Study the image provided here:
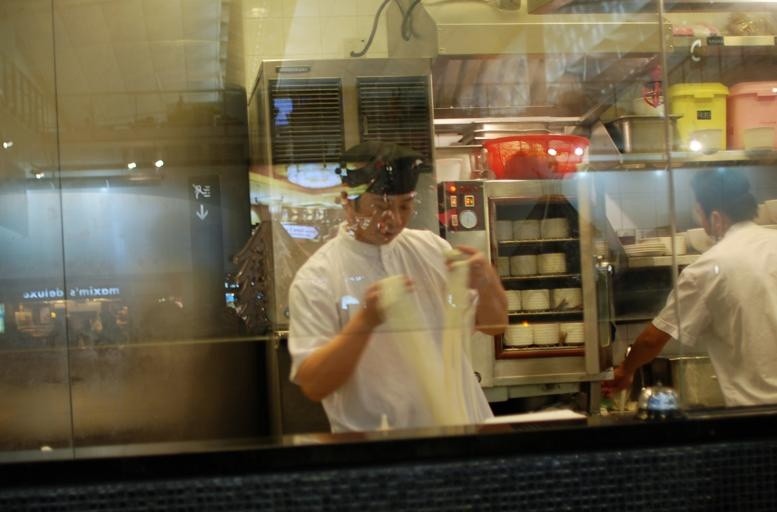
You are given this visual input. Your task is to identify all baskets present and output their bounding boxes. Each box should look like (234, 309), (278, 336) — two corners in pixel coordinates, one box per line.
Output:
(482, 135), (589, 181)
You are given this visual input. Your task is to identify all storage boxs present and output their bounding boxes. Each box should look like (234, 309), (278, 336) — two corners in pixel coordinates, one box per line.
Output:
(728, 79), (777, 150)
(667, 356), (726, 410)
(668, 81), (731, 151)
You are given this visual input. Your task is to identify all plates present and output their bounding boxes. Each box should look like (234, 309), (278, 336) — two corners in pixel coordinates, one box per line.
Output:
(622, 235), (687, 257)
(502, 321), (585, 348)
(492, 217), (572, 241)
(496, 252), (571, 277)
(505, 287), (583, 313)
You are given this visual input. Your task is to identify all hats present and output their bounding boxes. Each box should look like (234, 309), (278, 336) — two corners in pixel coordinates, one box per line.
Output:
(337, 142), (425, 195)
(690, 170), (750, 206)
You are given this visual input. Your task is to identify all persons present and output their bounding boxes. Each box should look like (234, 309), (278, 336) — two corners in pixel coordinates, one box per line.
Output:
(601, 169), (777, 409)
(287, 140), (509, 433)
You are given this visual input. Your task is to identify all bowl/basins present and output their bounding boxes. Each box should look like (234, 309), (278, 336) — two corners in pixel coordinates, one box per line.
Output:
(689, 128), (723, 154)
(674, 228), (718, 255)
(752, 199), (777, 225)
(741, 126), (776, 152)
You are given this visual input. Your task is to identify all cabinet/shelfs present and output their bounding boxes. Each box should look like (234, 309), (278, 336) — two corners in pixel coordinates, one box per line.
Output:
(589, 35), (777, 269)
